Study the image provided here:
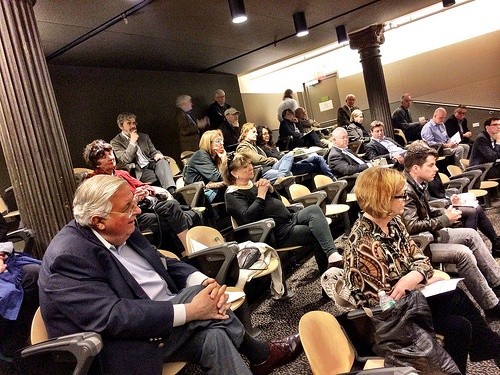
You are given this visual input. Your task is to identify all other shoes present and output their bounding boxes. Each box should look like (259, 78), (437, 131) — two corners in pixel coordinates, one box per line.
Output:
(469, 331), (500, 368)
(491, 284), (500, 299)
(492, 241), (500, 258)
(485, 301), (500, 323)
(327, 258), (344, 270)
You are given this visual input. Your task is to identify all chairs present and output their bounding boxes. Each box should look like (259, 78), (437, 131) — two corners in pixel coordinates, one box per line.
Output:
(1, 125), (500, 375)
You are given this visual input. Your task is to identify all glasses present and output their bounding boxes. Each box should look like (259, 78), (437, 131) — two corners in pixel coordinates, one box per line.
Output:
(102, 195), (139, 218)
(247, 131), (257, 133)
(391, 190), (407, 200)
(402, 100), (413, 102)
(456, 111), (466, 115)
(489, 124), (500, 126)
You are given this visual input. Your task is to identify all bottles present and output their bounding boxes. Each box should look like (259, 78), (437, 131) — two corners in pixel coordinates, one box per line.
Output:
(378, 290), (395, 313)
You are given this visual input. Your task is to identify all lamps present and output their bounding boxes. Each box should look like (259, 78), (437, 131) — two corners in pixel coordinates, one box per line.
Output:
(228, 0), (247, 24)
(442, 0), (455, 8)
(293, 11), (309, 38)
(336, 25), (349, 45)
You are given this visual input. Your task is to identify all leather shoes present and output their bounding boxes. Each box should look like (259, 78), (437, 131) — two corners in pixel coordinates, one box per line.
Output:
(249, 334), (304, 375)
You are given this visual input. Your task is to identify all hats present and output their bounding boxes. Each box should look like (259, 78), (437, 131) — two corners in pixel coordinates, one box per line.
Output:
(224, 108), (241, 116)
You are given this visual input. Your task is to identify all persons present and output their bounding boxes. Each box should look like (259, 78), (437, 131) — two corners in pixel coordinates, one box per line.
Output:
(83, 139), (190, 256)
(182, 130), (230, 203)
(344, 167), (499, 375)
(255, 125), (338, 182)
(275, 87), (500, 176)
(0, 213), (43, 362)
(39, 175), (305, 375)
(109, 114), (175, 195)
(236, 121), (297, 201)
(225, 151), (345, 277)
(327, 127), (375, 193)
(222, 107), (244, 152)
(173, 95), (207, 152)
(402, 141), (500, 323)
(206, 89), (231, 130)
